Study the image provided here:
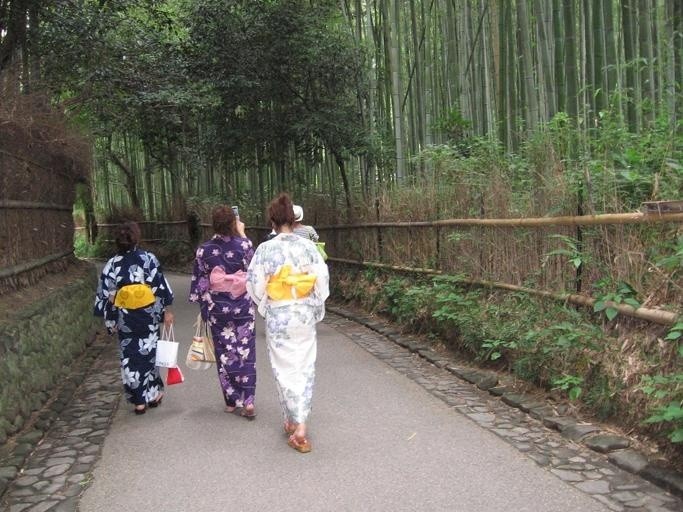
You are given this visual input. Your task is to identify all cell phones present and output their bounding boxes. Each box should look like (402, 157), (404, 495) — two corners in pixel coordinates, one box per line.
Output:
(231, 206), (239, 217)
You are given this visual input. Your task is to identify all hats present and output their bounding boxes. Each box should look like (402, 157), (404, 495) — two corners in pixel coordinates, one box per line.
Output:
(292, 205), (303, 222)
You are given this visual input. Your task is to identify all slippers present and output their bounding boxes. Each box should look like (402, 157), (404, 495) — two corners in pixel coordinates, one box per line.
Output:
(240, 409), (257, 417)
(288, 436), (311, 453)
(136, 395), (163, 414)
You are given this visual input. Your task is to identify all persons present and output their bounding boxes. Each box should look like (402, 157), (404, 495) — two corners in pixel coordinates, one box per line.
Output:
(293, 205), (319, 243)
(245, 191), (330, 453)
(188, 204), (257, 417)
(92, 220), (175, 414)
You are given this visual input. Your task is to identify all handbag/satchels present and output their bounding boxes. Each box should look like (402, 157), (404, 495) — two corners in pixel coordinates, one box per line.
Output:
(186, 337), (215, 370)
(155, 340), (184, 386)
(314, 242), (327, 261)
